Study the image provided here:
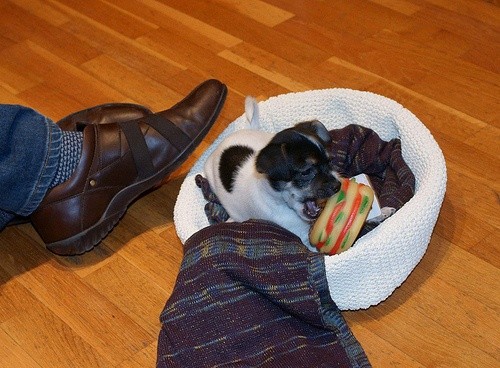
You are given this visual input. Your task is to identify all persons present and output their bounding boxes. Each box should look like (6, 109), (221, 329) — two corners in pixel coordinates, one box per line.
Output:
(0, 79), (228, 255)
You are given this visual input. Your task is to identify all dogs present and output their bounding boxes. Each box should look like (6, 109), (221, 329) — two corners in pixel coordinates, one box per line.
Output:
(205, 120), (341, 253)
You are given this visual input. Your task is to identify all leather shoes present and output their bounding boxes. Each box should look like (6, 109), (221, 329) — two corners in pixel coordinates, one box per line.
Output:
(7, 79), (228, 257)
